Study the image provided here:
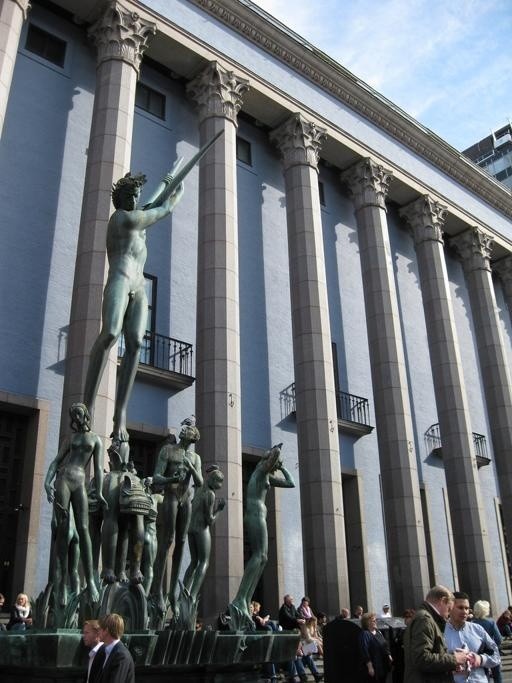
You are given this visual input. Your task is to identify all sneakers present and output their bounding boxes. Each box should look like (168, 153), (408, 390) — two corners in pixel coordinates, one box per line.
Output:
(315, 675), (323, 683)
(300, 675), (307, 683)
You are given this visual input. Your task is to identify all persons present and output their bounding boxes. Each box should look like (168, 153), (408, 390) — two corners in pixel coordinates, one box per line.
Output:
(67, 170), (188, 441)
(94, 612), (137, 682)
(247, 583), (512, 682)
(79, 618), (107, 682)
(40, 403), (298, 634)
(0, 593), (8, 631)
(4, 592), (33, 631)
